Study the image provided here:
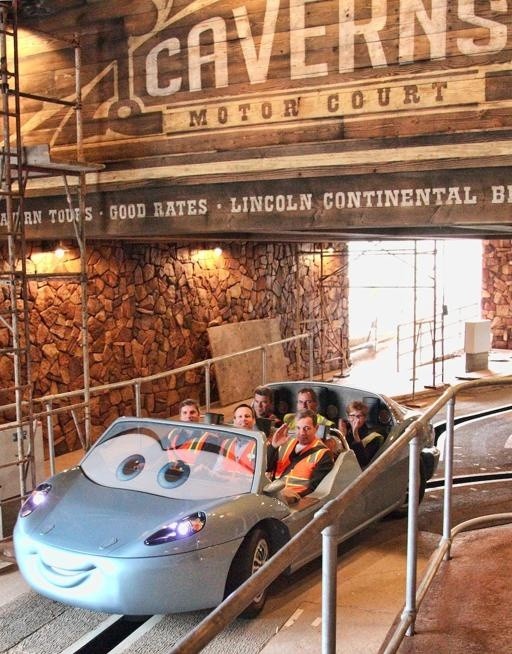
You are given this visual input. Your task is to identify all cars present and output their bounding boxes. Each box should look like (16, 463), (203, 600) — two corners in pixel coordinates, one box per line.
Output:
(14, 380), (440, 620)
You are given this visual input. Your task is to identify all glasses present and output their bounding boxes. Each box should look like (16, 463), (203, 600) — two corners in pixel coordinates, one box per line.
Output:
(348, 414), (366, 419)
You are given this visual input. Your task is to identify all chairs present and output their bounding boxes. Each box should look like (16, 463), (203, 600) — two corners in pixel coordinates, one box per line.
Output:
(266, 383), (283, 416)
(315, 423), (330, 444)
(203, 411), (229, 437)
(359, 394), (385, 438)
(255, 415), (278, 466)
(310, 382), (334, 421)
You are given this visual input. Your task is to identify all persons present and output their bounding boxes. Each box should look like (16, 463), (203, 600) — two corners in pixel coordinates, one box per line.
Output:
(161, 398), (219, 470)
(337, 401), (384, 469)
(287, 388), (338, 437)
(263, 409), (334, 504)
(252, 383), (283, 428)
(194, 404), (274, 482)
